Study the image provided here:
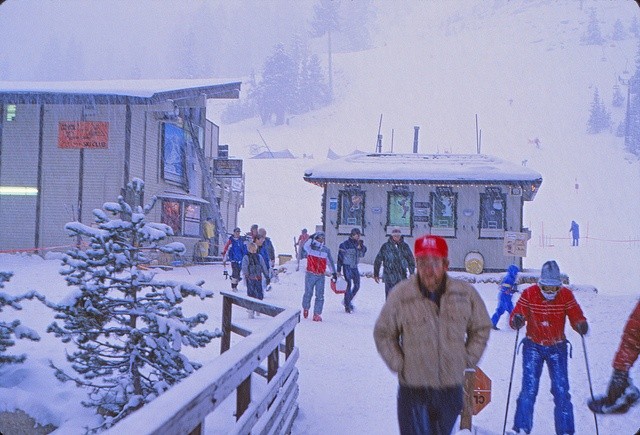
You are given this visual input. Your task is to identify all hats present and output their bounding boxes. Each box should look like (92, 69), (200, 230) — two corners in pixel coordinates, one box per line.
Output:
(414, 236), (448, 258)
(538, 261), (561, 286)
(312, 231), (325, 248)
(351, 229), (360, 235)
(391, 226), (401, 235)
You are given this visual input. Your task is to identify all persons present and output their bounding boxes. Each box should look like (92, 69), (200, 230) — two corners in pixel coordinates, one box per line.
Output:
(202, 217), (215, 256)
(221, 228), (247, 292)
(242, 243), (270, 316)
(337, 228), (367, 314)
(302, 231), (337, 322)
(373, 235), (492, 435)
(569, 221), (580, 246)
(252, 233), (269, 273)
(490, 265), (520, 330)
(602, 296), (640, 435)
(509, 261), (588, 435)
(374, 226), (416, 301)
(244, 224), (258, 243)
(296, 228), (309, 260)
(259, 228), (275, 291)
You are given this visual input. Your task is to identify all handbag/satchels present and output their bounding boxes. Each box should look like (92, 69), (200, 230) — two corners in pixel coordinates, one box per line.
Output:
(330, 276), (347, 293)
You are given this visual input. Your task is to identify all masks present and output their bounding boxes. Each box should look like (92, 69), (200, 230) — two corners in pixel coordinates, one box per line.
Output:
(541, 288), (560, 300)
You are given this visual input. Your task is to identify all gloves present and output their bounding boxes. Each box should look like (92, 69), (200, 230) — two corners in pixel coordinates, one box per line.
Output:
(609, 369), (629, 405)
(331, 273), (337, 284)
(578, 322), (588, 334)
(310, 234), (316, 239)
(512, 314), (525, 329)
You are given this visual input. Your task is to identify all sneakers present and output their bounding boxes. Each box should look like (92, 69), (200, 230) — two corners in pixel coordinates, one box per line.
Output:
(304, 310), (308, 318)
(343, 300), (353, 309)
(345, 307), (352, 312)
(313, 315), (322, 321)
(506, 426), (525, 435)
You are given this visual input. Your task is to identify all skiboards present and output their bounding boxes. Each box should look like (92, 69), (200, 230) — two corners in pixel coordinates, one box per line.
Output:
(294, 235), (301, 272)
